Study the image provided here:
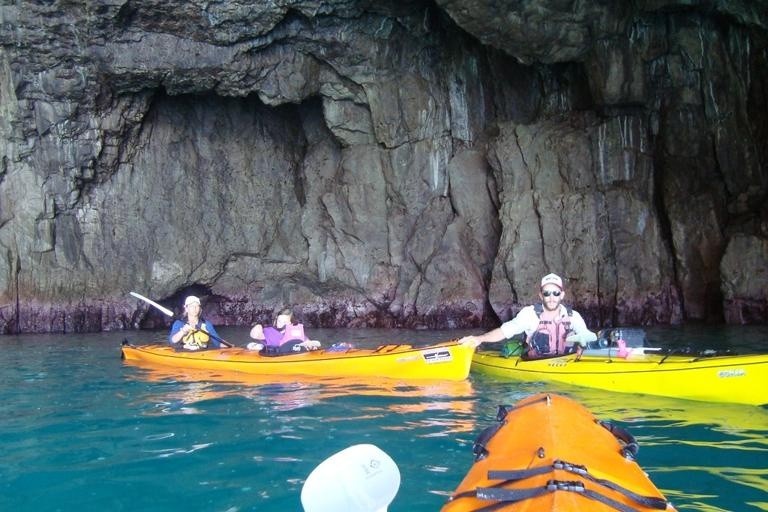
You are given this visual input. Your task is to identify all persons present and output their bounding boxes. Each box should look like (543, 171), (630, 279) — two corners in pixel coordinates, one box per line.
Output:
(457, 271), (596, 360)
(166, 295), (235, 350)
(250, 379), (318, 414)
(248, 309), (322, 355)
(127, 378), (240, 415)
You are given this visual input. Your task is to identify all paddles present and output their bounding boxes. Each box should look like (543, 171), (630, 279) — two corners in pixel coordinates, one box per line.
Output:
(302, 443), (400, 511)
(129, 291), (235, 348)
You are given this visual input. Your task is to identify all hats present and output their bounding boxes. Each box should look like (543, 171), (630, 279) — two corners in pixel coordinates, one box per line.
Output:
(541, 274), (563, 291)
(184, 296), (200, 307)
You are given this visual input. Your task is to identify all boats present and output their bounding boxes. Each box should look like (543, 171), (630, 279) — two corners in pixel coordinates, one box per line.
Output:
(119, 336), (478, 381)
(470, 349), (768, 408)
(438, 389), (676, 511)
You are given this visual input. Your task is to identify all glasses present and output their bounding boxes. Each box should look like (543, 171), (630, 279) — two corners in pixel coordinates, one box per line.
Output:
(543, 291), (560, 296)
